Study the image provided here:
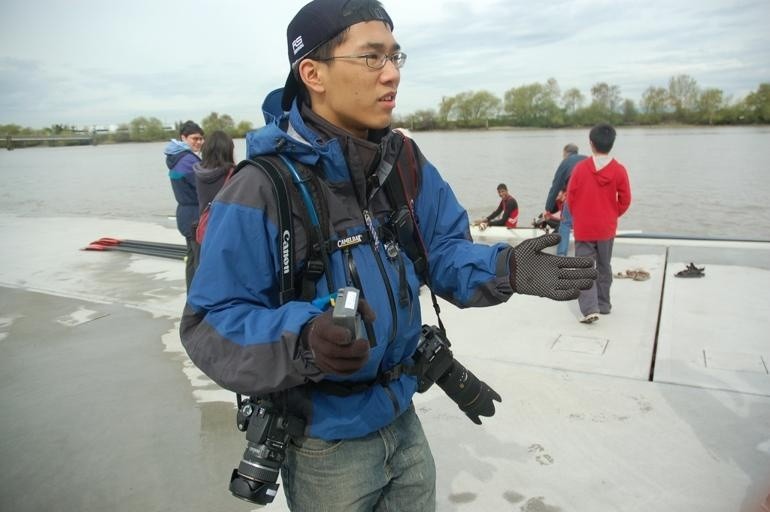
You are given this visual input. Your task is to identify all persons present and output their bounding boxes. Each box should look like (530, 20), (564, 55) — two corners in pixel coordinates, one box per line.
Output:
(163, 118), (208, 300)
(566, 124), (633, 324)
(176, 1), (600, 512)
(530, 190), (564, 232)
(543, 140), (587, 257)
(472, 184), (519, 231)
(190, 129), (239, 246)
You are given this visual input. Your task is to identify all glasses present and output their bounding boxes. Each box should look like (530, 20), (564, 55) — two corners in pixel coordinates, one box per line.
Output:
(308, 52), (406, 70)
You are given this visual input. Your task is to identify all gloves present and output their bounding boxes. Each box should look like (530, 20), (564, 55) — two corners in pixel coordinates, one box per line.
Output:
(300, 299), (376, 377)
(508, 234), (598, 301)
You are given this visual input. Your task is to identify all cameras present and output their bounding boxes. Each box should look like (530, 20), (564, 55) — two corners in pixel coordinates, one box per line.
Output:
(228, 399), (307, 505)
(332, 286), (362, 343)
(416, 324), (501, 425)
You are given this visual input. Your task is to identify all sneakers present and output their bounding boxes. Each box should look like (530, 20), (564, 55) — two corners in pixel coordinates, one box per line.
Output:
(579, 312), (600, 324)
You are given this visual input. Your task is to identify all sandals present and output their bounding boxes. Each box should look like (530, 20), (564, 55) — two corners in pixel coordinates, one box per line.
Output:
(633, 271), (650, 281)
(674, 263), (705, 278)
(613, 270), (637, 278)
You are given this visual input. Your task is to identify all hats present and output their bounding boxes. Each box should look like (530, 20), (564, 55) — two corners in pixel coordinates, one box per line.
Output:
(281, 0), (394, 112)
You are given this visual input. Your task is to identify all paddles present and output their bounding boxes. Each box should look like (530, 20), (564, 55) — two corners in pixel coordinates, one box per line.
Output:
(82, 237), (189, 262)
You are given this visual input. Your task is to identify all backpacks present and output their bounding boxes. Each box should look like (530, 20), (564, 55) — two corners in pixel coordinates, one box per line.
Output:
(187, 130), (423, 418)
